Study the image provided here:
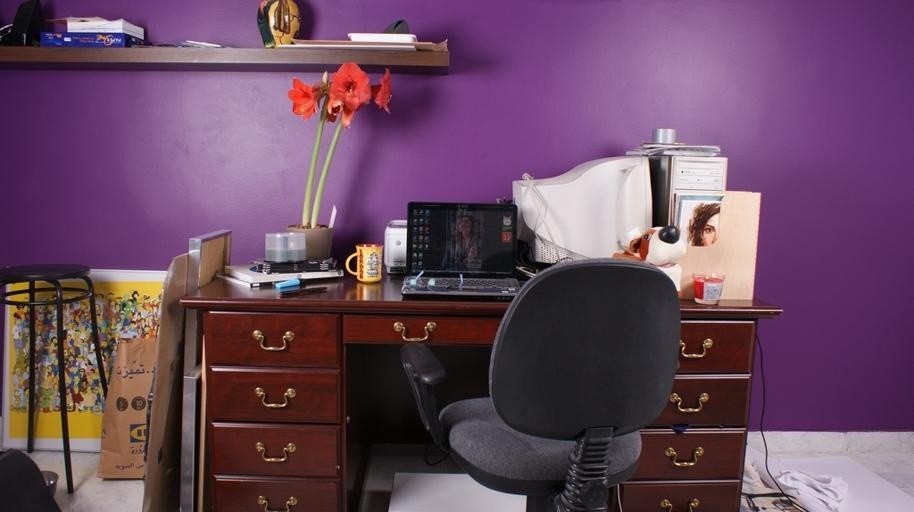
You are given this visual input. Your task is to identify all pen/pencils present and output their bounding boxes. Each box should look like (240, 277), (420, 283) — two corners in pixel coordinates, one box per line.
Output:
(278, 286), (330, 295)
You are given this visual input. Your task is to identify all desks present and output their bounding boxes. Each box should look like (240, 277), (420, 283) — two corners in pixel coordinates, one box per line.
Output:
(177, 257), (785, 511)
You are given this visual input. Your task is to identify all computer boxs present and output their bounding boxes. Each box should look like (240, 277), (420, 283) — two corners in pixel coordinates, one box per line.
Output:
(648, 155), (728, 227)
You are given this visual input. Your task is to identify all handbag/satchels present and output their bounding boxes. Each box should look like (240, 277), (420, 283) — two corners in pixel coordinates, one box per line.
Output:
(97, 338), (158, 481)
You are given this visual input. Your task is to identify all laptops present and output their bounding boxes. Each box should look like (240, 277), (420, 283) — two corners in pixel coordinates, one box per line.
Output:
(400, 201), (521, 301)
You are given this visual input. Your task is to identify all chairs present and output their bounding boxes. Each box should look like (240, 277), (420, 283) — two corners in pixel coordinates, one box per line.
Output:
(399, 260), (684, 508)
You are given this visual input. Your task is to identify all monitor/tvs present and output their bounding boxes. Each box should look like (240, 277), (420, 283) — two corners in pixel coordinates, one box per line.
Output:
(512, 155), (653, 264)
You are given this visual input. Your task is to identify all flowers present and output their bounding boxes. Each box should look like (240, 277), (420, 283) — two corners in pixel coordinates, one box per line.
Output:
(282, 60), (393, 226)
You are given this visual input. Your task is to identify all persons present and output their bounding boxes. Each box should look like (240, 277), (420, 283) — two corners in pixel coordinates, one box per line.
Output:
(12, 290), (162, 413)
(687, 200), (721, 247)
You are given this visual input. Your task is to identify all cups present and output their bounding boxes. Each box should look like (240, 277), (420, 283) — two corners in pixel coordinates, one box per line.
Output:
(346, 245), (384, 280)
(695, 270), (726, 305)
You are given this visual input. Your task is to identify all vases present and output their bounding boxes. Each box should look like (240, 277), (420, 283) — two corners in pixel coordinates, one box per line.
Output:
(290, 223), (334, 261)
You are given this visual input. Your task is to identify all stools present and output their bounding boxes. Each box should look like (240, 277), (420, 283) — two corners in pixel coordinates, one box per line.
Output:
(2, 262), (114, 495)
